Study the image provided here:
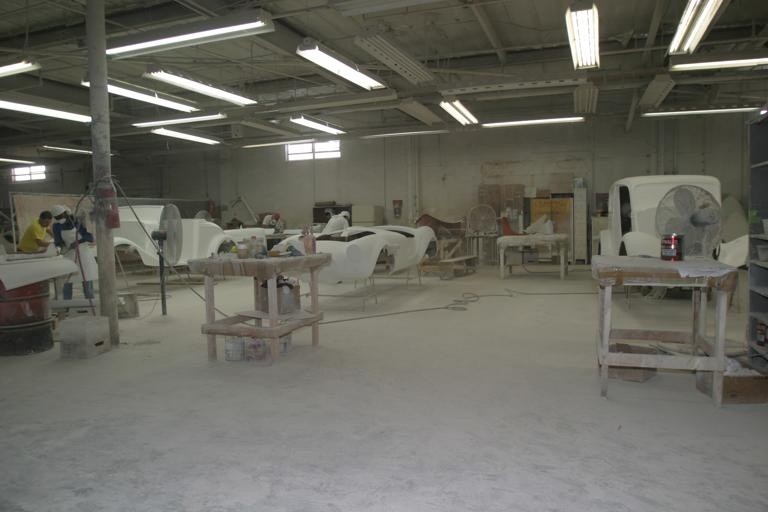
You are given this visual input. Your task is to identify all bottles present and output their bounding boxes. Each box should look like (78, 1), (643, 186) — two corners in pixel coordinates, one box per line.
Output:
(756, 325), (766, 346)
(238, 241), (248, 258)
(250, 236), (258, 258)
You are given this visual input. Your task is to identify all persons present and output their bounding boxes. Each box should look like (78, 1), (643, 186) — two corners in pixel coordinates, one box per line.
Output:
(17, 210), (53, 253)
(51, 204), (98, 299)
(322, 211), (350, 233)
(262, 213), (284, 229)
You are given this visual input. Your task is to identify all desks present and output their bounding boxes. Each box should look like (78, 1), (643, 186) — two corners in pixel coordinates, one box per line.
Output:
(590, 258), (736, 401)
(186, 251), (332, 362)
(496, 234), (570, 280)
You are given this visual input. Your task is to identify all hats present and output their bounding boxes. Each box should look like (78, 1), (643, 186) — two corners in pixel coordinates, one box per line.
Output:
(338, 210), (350, 218)
(51, 205), (65, 217)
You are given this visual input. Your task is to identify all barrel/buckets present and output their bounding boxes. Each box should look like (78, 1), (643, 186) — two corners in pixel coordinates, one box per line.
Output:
(257, 283), (301, 316)
(0, 268), (56, 357)
(224, 334), (244, 361)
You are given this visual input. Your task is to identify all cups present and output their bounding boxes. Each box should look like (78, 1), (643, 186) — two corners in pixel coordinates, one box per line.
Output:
(756, 245), (768, 263)
(761, 218), (768, 236)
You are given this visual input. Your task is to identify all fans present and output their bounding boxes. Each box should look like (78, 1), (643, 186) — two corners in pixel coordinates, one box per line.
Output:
(150, 202), (183, 329)
(655, 184), (723, 353)
(470, 204), (497, 264)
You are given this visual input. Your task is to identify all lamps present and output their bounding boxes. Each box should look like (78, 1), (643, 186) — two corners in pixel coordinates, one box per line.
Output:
(0, 7), (277, 163)
(569, 81), (600, 117)
(287, 112), (349, 139)
(668, 47), (767, 73)
(293, 35), (386, 97)
(399, 95), (445, 130)
(637, 72), (675, 111)
(481, 110), (588, 130)
(356, 123), (455, 138)
(352, 25), (437, 87)
(640, 100), (762, 120)
(665, 1), (728, 59)
(563, 0), (606, 73)
(436, 92), (480, 128)
(238, 115), (303, 142)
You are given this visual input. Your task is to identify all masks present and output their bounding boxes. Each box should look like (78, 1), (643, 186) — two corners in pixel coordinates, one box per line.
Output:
(57, 218), (67, 224)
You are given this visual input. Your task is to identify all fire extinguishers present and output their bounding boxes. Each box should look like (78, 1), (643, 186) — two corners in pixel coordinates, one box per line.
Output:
(100, 174), (121, 229)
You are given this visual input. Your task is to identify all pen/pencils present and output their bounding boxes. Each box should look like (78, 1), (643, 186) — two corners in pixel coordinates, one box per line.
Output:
(303, 224), (315, 234)
